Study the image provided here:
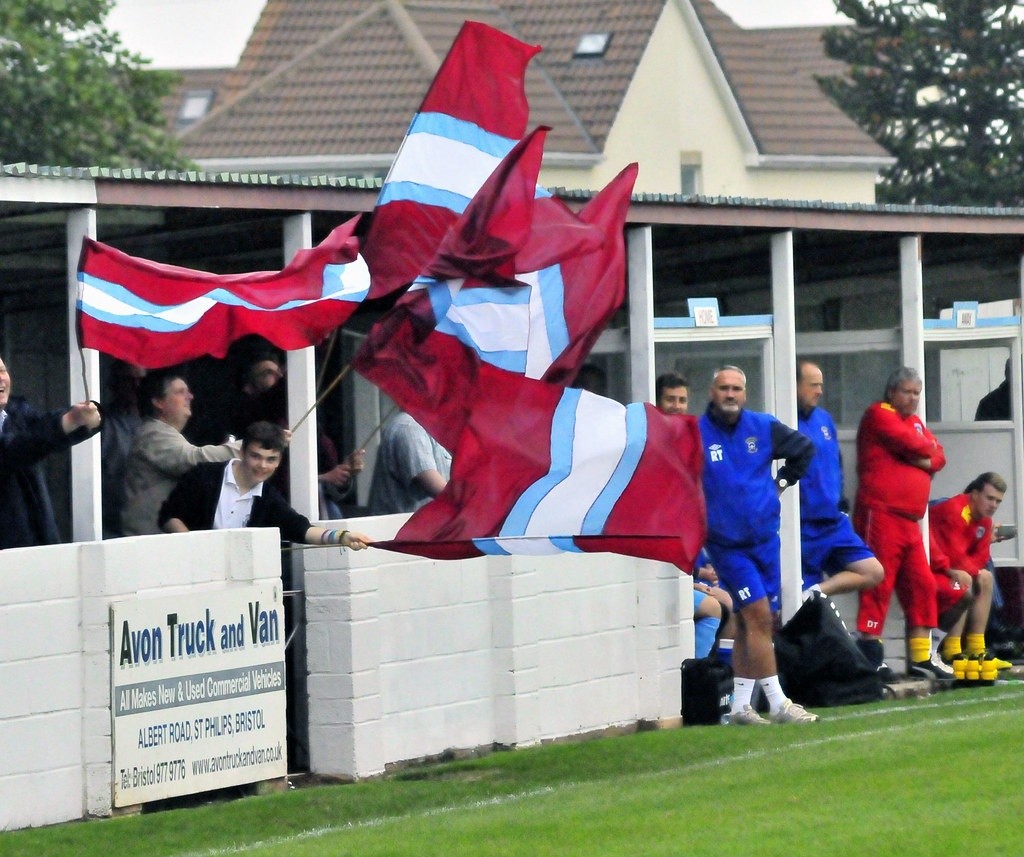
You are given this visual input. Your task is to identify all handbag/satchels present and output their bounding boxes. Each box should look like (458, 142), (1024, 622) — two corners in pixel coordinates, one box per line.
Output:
(679, 655), (733, 722)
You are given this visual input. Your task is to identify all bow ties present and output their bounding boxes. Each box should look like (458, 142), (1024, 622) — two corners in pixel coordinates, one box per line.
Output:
(935, 646), (987, 674)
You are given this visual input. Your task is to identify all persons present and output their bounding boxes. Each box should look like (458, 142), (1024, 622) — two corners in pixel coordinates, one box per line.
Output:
(100, 360), (456, 551)
(0, 357), (103, 551)
(973, 354), (1024, 649)
(649, 360), (1012, 727)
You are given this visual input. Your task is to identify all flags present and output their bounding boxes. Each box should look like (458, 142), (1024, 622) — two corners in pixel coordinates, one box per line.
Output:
(341, 21), (706, 575)
(74, 213), (370, 368)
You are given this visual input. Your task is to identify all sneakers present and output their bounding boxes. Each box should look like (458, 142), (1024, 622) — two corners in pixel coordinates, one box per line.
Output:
(768, 700), (818, 724)
(904, 650), (955, 678)
(719, 705), (771, 727)
(865, 657), (901, 684)
(970, 653), (1013, 672)
